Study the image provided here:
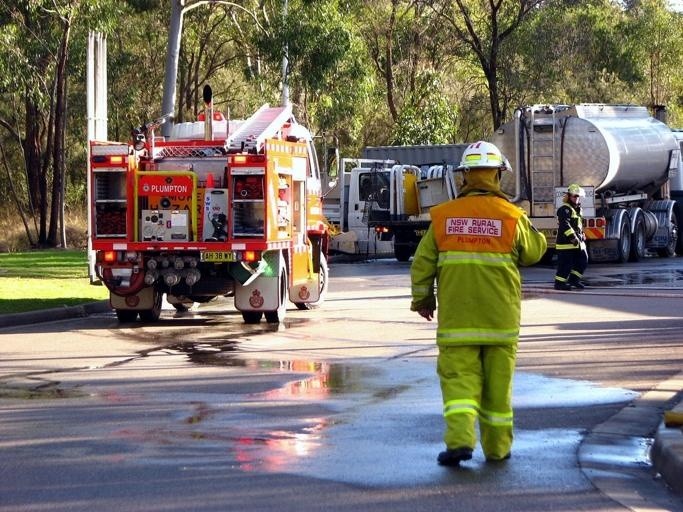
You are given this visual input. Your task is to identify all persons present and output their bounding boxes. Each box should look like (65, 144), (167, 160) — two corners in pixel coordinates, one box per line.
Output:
(408, 140), (549, 464)
(553, 183), (589, 290)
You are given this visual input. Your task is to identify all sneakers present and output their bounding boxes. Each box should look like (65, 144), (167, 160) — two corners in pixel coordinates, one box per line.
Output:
(568, 279), (585, 289)
(486, 450), (511, 464)
(554, 283), (574, 290)
(438, 446), (473, 462)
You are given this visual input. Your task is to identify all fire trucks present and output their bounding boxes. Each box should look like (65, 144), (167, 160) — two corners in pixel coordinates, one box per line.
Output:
(78, 81), (336, 327)
(486, 101), (683, 264)
(320, 143), (475, 263)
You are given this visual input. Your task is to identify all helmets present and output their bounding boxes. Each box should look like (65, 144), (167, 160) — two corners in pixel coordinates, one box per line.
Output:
(452, 140), (503, 171)
(569, 185), (585, 196)
